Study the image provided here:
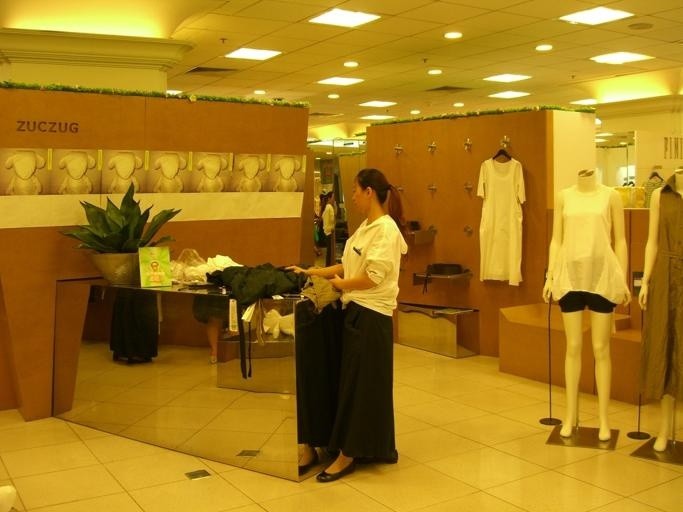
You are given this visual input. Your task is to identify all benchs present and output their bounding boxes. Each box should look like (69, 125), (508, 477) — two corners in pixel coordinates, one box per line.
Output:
(215, 326), (297, 397)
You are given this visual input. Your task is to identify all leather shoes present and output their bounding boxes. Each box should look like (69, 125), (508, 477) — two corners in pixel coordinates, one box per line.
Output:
(316, 459), (355, 482)
(298, 450), (319, 475)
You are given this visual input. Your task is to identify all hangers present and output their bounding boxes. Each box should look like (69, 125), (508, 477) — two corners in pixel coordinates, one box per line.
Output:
(494, 137), (511, 160)
(647, 165), (662, 182)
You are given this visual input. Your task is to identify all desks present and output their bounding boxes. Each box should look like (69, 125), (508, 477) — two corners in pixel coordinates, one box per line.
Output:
(51, 277), (336, 482)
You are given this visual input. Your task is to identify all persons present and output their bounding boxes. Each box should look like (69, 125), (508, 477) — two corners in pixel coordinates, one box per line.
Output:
(542, 169), (632, 442)
(294, 298), (342, 475)
(638, 168), (683, 453)
(321, 192), (335, 267)
(285, 168), (410, 483)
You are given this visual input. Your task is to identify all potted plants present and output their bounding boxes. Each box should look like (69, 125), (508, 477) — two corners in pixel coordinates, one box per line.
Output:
(57, 182), (182, 286)
